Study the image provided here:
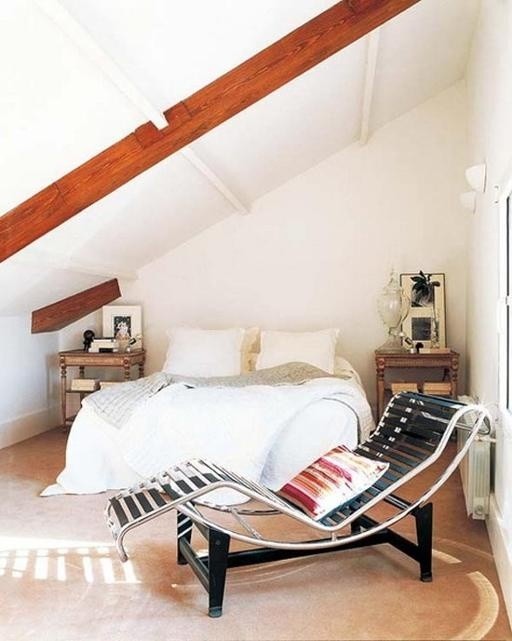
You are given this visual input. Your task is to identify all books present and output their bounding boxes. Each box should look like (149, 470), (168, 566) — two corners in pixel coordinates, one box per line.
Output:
(387, 379), (452, 396)
(419, 348), (450, 354)
(71, 377), (126, 392)
(88, 337), (131, 353)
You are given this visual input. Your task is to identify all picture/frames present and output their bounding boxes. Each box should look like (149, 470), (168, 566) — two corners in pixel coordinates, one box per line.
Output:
(102, 305), (143, 354)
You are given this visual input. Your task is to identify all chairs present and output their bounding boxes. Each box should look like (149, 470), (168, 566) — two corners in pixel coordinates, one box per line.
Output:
(102, 389), (487, 620)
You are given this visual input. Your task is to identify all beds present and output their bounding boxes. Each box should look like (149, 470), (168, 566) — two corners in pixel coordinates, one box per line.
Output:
(36, 347), (375, 506)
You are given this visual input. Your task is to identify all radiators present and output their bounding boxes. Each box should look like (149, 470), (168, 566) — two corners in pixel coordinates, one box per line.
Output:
(457, 396), (492, 523)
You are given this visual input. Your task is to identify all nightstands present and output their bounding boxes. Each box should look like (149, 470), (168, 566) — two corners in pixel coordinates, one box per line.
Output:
(374, 345), (458, 435)
(58, 349), (148, 438)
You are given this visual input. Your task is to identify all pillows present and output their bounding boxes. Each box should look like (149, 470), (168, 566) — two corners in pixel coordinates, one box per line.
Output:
(257, 329), (340, 376)
(159, 327), (256, 380)
(276, 443), (390, 520)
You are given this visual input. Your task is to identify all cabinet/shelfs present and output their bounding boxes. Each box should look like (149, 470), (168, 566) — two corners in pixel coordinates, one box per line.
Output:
(399, 272), (446, 350)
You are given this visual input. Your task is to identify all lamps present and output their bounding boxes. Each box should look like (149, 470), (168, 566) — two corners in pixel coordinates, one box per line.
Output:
(457, 189), (477, 214)
(463, 162), (487, 195)
